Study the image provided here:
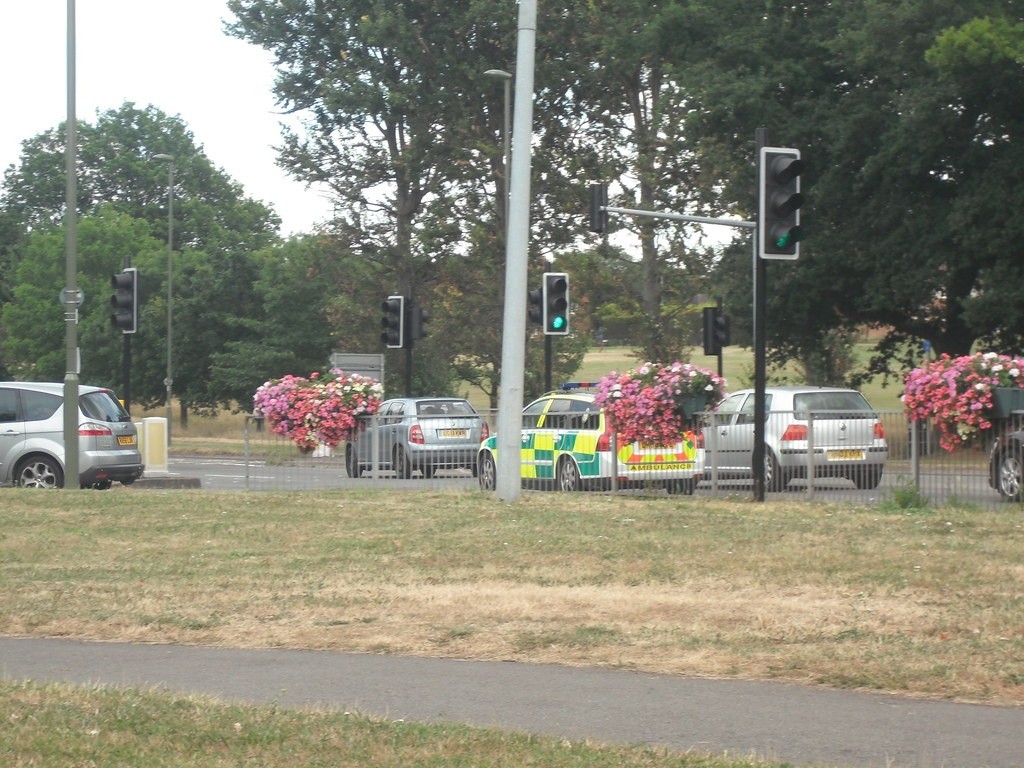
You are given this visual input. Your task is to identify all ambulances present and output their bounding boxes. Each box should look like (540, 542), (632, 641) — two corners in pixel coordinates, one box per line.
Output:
(475, 382), (706, 492)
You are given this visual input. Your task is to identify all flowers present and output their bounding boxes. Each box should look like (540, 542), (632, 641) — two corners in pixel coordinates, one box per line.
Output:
(899, 350), (1024, 453)
(593, 358), (727, 450)
(252, 367), (386, 454)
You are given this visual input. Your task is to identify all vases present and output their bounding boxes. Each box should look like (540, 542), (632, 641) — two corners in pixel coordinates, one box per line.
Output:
(979, 386), (1024, 419)
(671, 393), (707, 419)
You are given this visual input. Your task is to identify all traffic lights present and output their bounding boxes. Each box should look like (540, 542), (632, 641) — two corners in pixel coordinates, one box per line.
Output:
(543, 272), (569, 335)
(757, 146), (805, 260)
(110, 267), (138, 335)
(714, 314), (730, 346)
(381, 296), (405, 349)
(527, 288), (543, 326)
(589, 183), (608, 233)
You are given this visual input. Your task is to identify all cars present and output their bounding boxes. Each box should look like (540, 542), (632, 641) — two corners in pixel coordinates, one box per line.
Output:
(700, 386), (889, 492)
(345, 397), (489, 479)
(0, 381), (145, 489)
(988, 424), (1024, 502)
